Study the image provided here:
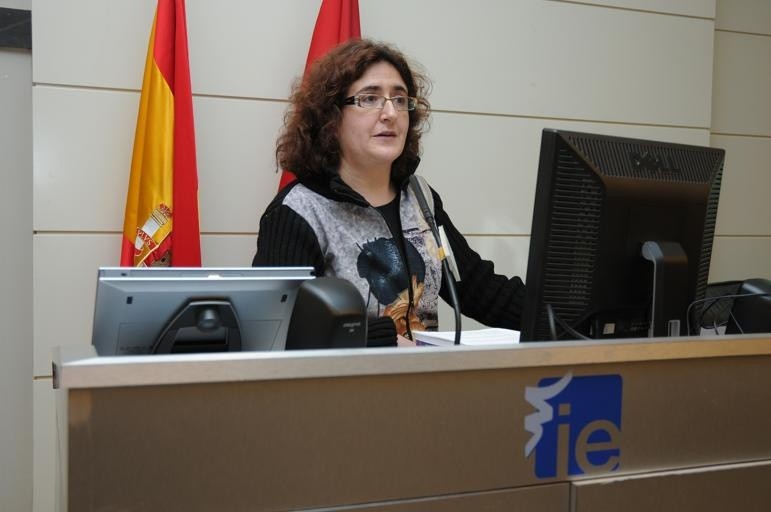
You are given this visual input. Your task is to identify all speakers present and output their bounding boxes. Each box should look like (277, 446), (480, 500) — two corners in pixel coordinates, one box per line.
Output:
(285, 278), (366, 349)
(728, 278), (771, 334)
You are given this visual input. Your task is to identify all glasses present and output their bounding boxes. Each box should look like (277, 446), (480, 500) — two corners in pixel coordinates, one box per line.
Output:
(335, 92), (418, 113)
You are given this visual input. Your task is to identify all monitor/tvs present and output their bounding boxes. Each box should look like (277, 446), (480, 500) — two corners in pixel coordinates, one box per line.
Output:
(518, 129), (726, 345)
(90, 265), (312, 358)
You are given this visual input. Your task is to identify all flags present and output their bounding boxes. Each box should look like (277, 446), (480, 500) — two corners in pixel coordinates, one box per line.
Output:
(277, 1), (360, 193)
(118, 0), (201, 267)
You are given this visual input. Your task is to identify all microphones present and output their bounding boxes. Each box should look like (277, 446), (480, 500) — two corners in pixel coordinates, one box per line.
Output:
(410, 173), (461, 344)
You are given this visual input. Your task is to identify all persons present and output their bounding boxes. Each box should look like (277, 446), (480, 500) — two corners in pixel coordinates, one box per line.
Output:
(250, 38), (525, 341)
